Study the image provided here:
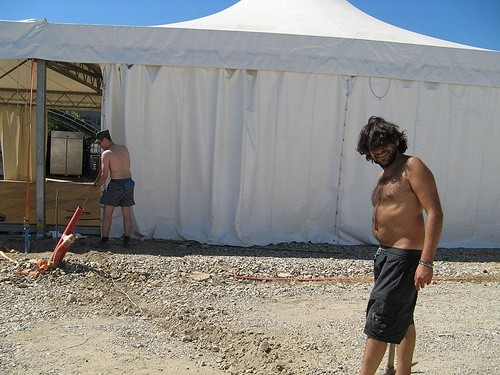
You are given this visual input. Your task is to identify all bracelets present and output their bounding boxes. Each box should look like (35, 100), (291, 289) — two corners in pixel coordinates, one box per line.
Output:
(419, 260), (436, 269)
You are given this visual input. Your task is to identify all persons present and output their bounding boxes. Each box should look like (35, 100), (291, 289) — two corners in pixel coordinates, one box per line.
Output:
(90, 127), (137, 244)
(353, 116), (446, 375)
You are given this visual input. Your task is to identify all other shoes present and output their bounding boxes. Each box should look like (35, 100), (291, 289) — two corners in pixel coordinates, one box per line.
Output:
(120, 234), (130, 246)
(96, 236), (108, 244)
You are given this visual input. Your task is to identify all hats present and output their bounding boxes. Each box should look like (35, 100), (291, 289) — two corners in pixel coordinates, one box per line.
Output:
(94, 129), (109, 143)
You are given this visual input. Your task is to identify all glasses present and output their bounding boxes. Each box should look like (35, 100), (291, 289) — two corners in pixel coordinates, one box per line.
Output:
(98, 140), (102, 145)
